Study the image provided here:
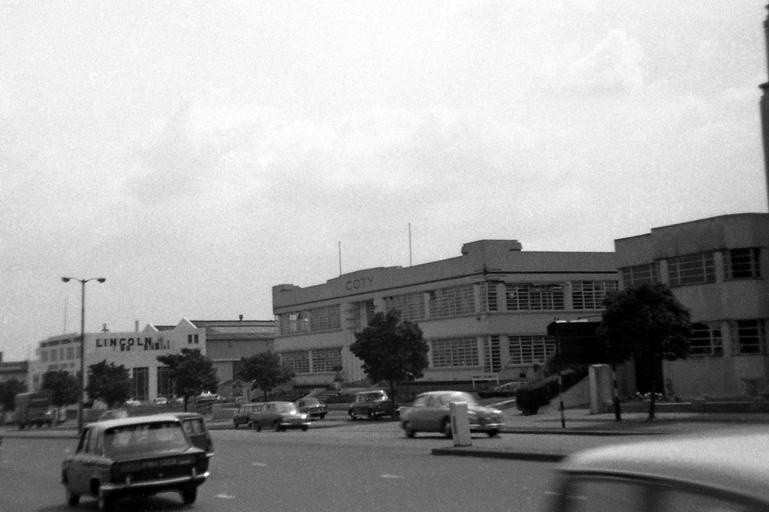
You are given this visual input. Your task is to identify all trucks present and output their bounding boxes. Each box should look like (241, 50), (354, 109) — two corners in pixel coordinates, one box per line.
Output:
(14, 391), (54, 429)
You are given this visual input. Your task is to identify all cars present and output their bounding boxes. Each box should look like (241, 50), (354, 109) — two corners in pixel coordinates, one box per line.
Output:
(233, 397), (327, 432)
(400, 391), (503, 438)
(349, 390), (391, 418)
(479, 381), (527, 398)
(60, 390), (227, 510)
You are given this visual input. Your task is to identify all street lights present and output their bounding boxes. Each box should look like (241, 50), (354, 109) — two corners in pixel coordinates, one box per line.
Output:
(62, 277), (105, 432)
